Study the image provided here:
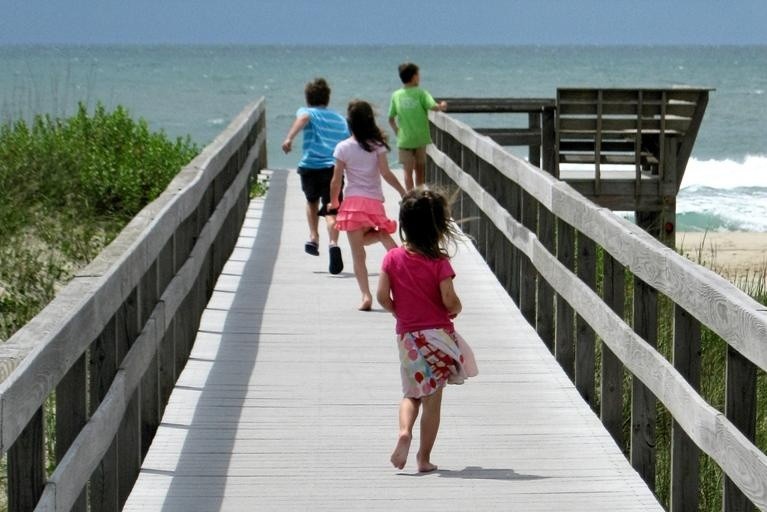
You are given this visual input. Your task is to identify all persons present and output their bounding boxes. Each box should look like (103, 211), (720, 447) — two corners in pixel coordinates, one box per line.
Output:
(389, 62), (448, 190)
(376, 184), (479, 472)
(282, 78), (351, 275)
(327, 101), (408, 311)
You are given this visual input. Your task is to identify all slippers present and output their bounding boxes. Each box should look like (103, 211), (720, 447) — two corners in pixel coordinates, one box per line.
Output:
(304, 241), (319, 256)
(328, 245), (344, 274)
